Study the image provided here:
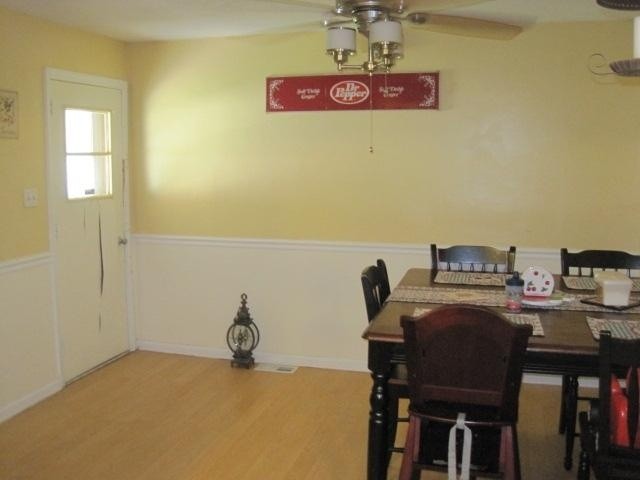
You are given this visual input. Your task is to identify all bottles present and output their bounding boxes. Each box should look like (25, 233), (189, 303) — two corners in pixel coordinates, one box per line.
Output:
(505, 272), (523, 313)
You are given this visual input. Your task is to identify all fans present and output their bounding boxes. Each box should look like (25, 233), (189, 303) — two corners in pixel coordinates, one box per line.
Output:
(223, 0), (524, 45)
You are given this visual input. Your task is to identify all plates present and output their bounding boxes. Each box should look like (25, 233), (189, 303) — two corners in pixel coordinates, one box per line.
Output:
(579, 295), (639, 310)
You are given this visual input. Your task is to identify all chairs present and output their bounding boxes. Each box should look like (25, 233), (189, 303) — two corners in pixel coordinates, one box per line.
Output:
(578, 329), (640, 480)
(362, 259), (414, 454)
(431, 244), (515, 284)
(399, 302), (534, 480)
(559, 247), (639, 471)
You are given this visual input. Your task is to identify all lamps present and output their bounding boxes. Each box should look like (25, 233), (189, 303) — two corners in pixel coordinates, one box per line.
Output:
(586, 15), (639, 77)
(226, 292), (260, 370)
(323, 0), (407, 153)
